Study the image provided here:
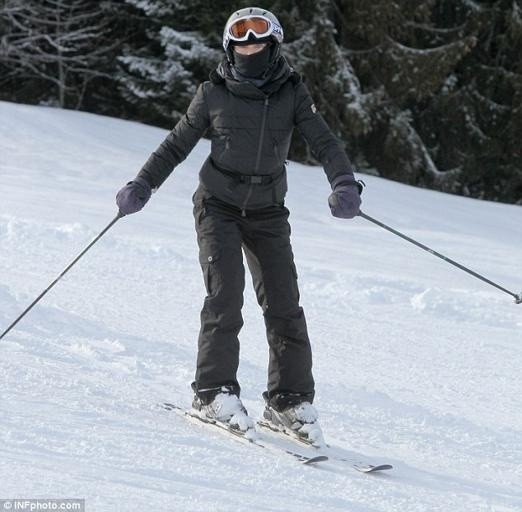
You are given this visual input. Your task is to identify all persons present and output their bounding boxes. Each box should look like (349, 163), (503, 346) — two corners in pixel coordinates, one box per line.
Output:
(116, 7), (365, 447)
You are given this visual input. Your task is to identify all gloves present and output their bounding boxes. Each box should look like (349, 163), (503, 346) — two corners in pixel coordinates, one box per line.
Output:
(327, 182), (363, 219)
(115, 177), (151, 216)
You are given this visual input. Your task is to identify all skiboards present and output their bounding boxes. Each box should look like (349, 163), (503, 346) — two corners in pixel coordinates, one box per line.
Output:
(161, 403), (392, 473)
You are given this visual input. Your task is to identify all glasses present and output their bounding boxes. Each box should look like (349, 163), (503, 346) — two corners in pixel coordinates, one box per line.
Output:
(224, 17), (282, 48)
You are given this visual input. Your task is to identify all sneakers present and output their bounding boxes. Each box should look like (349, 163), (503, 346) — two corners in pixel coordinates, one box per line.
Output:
(264, 397), (326, 448)
(190, 385), (255, 437)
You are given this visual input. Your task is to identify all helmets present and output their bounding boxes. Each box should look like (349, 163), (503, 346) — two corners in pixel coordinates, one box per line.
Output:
(221, 6), (283, 63)
